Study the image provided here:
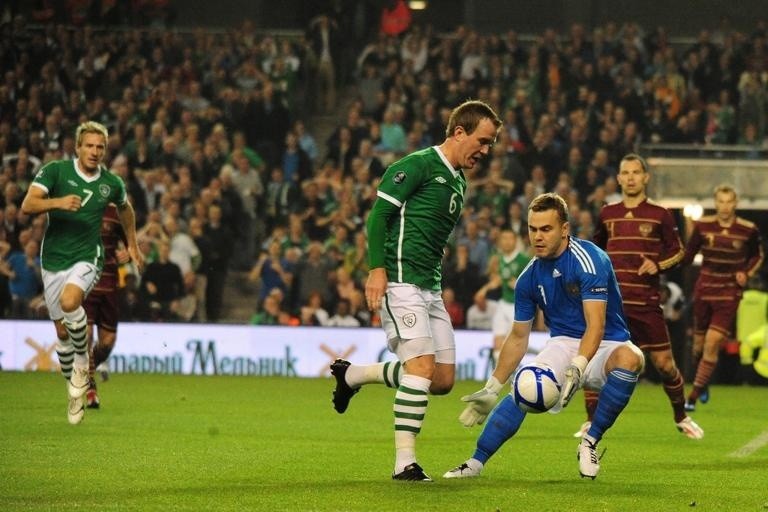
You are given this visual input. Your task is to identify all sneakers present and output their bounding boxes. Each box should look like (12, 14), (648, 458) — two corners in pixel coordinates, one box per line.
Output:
(674, 390), (708, 439)
(442, 463), (479, 479)
(391, 464), (433, 483)
(573, 422), (599, 480)
(67, 362), (99, 425)
(329, 359), (361, 413)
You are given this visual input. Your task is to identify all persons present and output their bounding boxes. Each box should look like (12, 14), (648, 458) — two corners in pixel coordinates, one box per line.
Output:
(442, 192), (646, 480)
(81, 202), (133, 408)
(21, 121), (142, 424)
(573, 154), (703, 440)
(684, 185), (765, 410)
(1, 0), (768, 382)
(329, 100), (503, 483)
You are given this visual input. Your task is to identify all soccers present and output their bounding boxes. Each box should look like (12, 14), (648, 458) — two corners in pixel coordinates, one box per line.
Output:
(512, 363), (560, 412)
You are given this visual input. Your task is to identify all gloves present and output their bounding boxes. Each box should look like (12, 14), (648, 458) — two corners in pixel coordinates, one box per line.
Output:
(458, 376), (505, 428)
(546, 356), (588, 414)
